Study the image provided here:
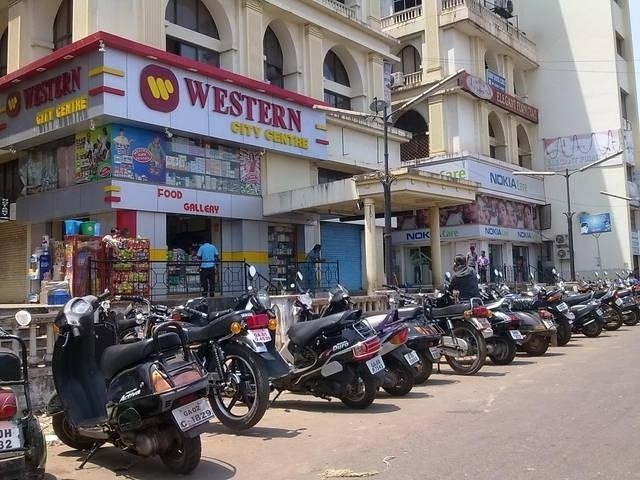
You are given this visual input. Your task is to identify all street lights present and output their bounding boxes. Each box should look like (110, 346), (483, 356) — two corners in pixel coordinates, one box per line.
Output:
(313, 69), (467, 285)
(513, 150), (639, 280)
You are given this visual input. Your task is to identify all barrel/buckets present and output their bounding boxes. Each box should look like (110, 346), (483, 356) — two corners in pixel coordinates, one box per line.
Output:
(64, 219), (81, 235)
(81, 221), (96, 235)
(47, 290), (70, 305)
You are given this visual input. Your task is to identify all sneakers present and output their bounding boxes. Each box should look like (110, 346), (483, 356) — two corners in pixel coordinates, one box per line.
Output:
(202, 292), (214, 297)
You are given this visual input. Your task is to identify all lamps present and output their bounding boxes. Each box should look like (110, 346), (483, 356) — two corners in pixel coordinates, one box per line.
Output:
(164, 128), (173, 138)
(259, 149), (266, 157)
(9, 145), (17, 154)
(98, 39), (106, 53)
(88, 119), (95, 130)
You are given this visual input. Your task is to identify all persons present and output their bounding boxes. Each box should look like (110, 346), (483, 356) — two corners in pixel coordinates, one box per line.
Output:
(148, 135), (166, 174)
(84, 128), (111, 170)
(303, 244), (325, 295)
(466, 246), (478, 269)
(196, 236), (220, 297)
(113, 128), (133, 144)
(101, 227), (121, 242)
(449, 254), (482, 304)
(397, 195), (534, 230)
(477, 250), (489, 284)
(246, 153), (258, 173)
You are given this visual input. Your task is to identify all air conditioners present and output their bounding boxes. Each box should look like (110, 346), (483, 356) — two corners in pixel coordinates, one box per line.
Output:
(390, 71), (404, 87)
(555, 233), (570, 260)
(494, 0), (514, 18)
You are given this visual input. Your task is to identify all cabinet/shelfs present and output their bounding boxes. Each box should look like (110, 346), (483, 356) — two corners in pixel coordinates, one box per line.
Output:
(164, 134), (241, 194)
(106, 238), (150, 301)
(268, 225), (298, 290)
(27, 247), (44, 304)
(168, 247), (202, 294)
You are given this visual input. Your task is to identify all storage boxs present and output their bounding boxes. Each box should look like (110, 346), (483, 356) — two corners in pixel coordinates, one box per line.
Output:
(64, 220), (101, 236)
